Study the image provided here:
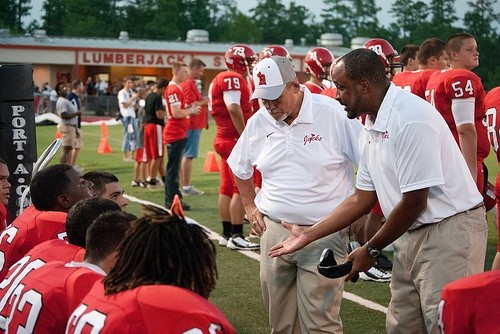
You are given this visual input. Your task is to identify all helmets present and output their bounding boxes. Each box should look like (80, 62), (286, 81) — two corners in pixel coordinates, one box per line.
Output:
(364, 39), (398, 67)
(304, 48), (336, 79)
(224, 45), (258, 73)
(259, 45), (293, 62)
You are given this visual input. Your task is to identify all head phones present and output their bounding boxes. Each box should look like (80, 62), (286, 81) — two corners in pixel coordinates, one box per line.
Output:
(317, 241), (362, 283)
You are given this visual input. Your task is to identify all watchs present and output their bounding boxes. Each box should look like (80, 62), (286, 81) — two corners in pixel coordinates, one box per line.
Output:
(364, 241), (381, 259)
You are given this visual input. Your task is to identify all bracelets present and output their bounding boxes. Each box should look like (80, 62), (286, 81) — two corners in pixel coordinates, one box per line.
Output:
(188, 106), (195, 114)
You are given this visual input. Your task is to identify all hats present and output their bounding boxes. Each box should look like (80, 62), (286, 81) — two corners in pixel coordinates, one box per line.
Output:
(250, 56), (294, 101)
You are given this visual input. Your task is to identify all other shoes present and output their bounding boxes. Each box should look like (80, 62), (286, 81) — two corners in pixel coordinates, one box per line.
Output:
(166, 201), (190, 210)
(147, 181), (165, 192)
(181, 187), (204, 196)
(73, 165), (83, 172)
(124, 157), (136, 162)
(139, 180), (148, 187)
(131, 180), (140, 186)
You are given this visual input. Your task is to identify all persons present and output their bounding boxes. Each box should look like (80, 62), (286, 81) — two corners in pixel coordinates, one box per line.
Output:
(268, 47), (485, 334)
(163, 63), (202, 209)
(68, 80), (85, 172)
(34, 76), (157, 116)
(481, 86), (500, 270)
(206, 33), (496, 282)
(226, 56), (365, 334)
(118, 75), (169, 190)
(181, 60), (210, 195)
(437, 269), (500, 334)
(0, 158), (236, 334)
(55, 82), (81, 167)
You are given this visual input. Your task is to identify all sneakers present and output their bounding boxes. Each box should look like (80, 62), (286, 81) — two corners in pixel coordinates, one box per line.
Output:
(227, 235), (260, 250)
(218, 235), (228, 246)
(359, 266), (392, 282)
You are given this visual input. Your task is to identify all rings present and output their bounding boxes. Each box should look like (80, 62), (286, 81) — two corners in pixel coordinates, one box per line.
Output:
(252, 220), (259, 226)
